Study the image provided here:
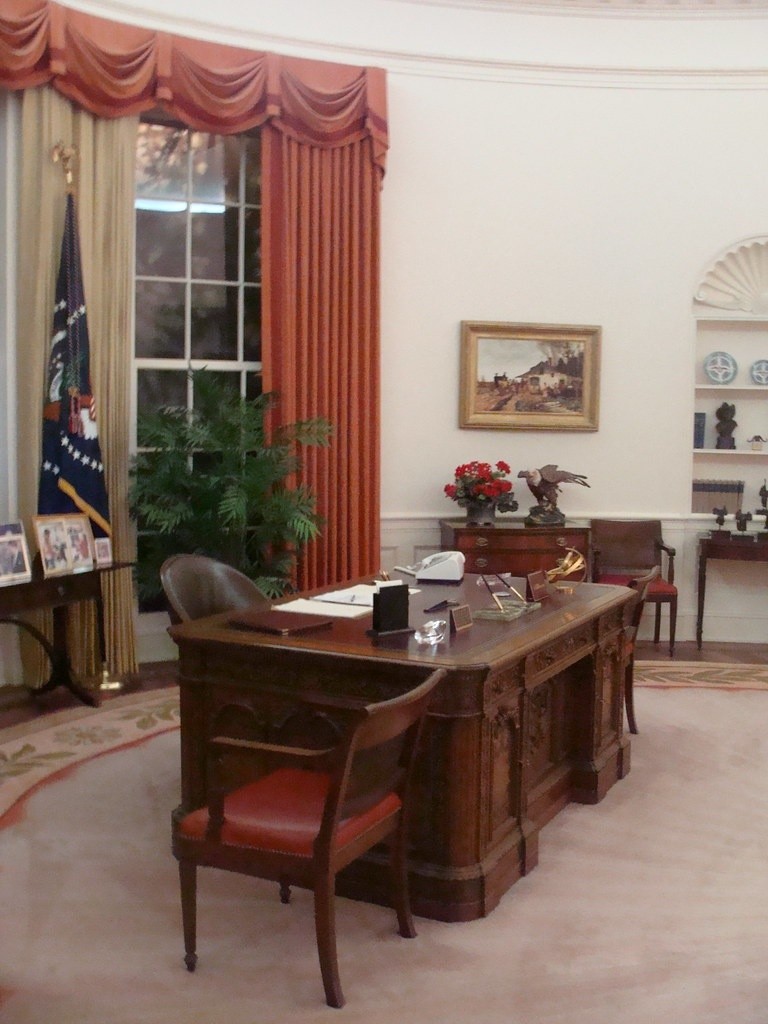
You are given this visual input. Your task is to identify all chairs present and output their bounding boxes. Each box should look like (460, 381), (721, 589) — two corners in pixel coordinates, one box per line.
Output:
(588, 518), (677, 659)
(625, 565), (659, 734)
(174, 668), (446, 1009)
(159, 553), (271, 626)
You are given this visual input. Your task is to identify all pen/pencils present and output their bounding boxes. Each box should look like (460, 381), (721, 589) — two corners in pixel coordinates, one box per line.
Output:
(481, 574), (502, 610)
(351, 594), (356, 602)
(496, 574), (526, 603)
(379, 570), (391, 581)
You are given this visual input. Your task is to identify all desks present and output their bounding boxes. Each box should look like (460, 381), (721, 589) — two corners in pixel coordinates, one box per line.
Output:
(0, 560), (138, 709)
(165, 564), (643, 923)
(695, 531), (768, 653)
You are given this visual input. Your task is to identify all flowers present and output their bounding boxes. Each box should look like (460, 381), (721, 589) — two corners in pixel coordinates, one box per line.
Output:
(444, 460), (519, 514)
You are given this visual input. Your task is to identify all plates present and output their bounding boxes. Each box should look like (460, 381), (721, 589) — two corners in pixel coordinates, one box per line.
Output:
(702, 351), (738, 385)
(750, 360), (768, 385)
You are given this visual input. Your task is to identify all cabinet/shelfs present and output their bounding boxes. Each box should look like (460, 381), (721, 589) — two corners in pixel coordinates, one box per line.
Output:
(691, 385), (768, 518)
(439, 519), (590, 583)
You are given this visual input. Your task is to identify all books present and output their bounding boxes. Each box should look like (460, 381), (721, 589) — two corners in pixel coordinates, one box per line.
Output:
(226, 608), (334, 636)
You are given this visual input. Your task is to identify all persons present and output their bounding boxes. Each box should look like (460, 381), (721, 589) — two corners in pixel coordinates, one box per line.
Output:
(74, 533), (88, 559)
(44, 529), (68, 571)
(6, 540), (26, 573)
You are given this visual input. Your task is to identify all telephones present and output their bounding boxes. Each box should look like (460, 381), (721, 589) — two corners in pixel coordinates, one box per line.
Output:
(415, 550), (466, 585)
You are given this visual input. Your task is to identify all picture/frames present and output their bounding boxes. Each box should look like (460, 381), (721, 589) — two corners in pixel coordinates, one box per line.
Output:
(457, 319), (602, 433)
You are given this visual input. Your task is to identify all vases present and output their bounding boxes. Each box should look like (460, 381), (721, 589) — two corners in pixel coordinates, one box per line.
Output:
(464, 497), (496, 528)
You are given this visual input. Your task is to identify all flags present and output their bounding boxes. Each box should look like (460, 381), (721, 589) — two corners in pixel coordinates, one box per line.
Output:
(36, 193), (112, 566)
